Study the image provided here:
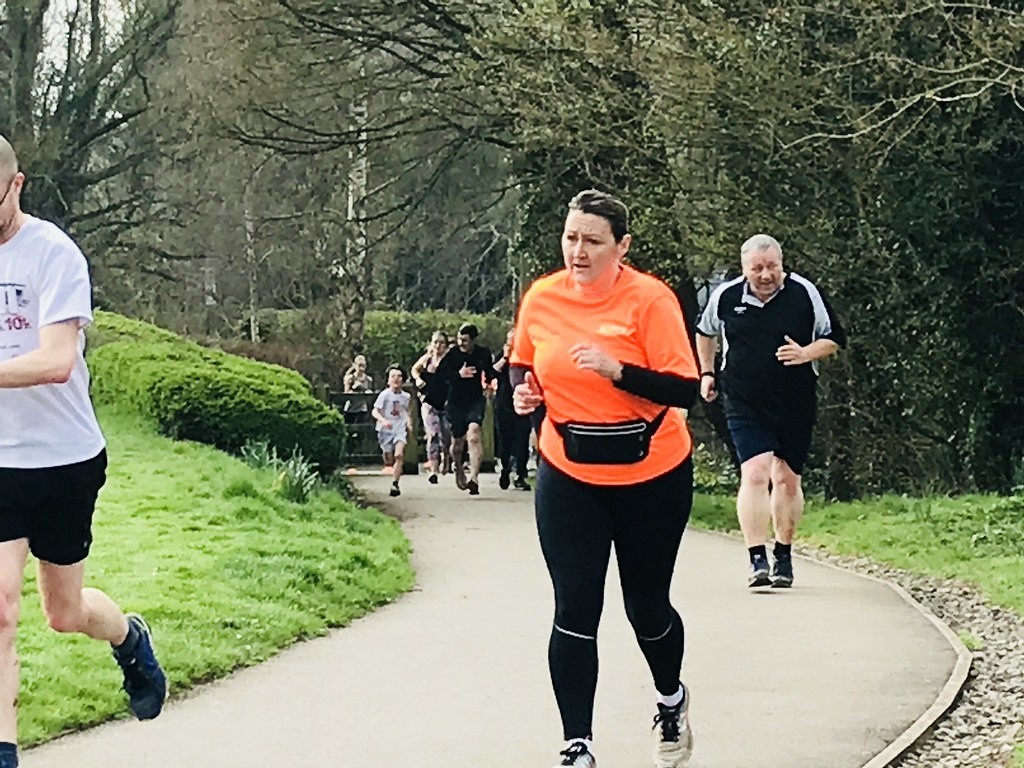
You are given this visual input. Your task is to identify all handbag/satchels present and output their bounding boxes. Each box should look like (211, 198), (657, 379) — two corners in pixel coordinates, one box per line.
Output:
(548, 405), (671, 464)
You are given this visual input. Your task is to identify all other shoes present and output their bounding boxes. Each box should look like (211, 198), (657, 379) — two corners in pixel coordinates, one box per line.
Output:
(389, 486), (401, 496)
(513, 477), (532, 491)
(454, 468), (468, 491)
(427, 473), (439, 484)
(500, 469), (512, 489)
(466, 479), (480, 495)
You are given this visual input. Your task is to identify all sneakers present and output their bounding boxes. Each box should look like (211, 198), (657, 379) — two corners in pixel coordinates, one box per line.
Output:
(650, 680), (697, 768)
(747, 554), (774, 592)
(553, 741), (598, 768)
(770, 554), (794, 587)
(109, 611), (170, 721)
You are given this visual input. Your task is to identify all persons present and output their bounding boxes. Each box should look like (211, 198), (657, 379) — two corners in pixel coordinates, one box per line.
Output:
(344, 322), (544, 497)
(509, 189), (700, 768)
(0, 135), (174, 768)
(695, 233), (847, 592)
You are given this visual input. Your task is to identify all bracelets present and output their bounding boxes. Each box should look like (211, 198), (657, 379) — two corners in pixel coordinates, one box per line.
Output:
(701, 370), (716, 378)
